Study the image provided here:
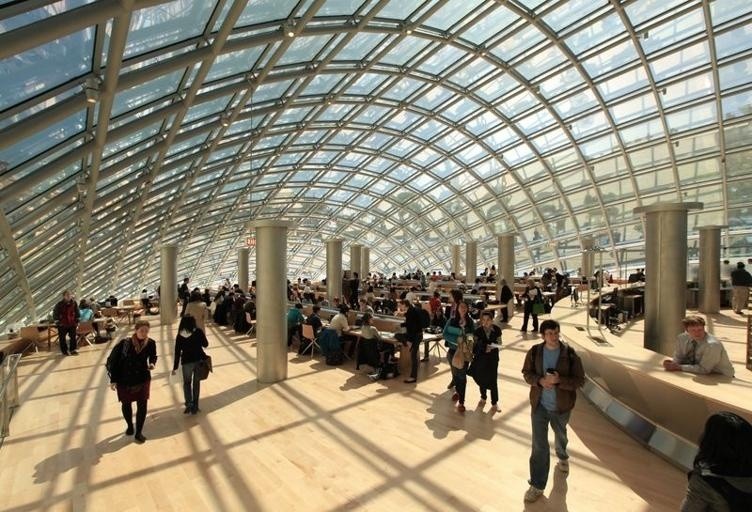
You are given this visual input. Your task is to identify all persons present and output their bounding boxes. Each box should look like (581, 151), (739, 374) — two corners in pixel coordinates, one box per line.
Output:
(53, 290), (80, 356)
(473, 310), (501, 412)
(664, 262), (751, 378)
(172, 313), (208, 415)
(443, 300), (475, 412)
(521, 320), (585, 502)
(110, 320), (158, 442)
(288, 265), (514, 384)
(79, 284), (160, 338)
(519, 265), (645, 332)
(679, 412), (751, 512)
(178, 277), (256, 336)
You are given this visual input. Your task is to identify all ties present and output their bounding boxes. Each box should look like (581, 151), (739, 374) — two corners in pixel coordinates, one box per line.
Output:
(688, 341), (697, 364)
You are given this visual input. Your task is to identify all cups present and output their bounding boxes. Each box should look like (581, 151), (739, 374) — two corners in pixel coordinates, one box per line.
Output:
(545, 368), (556, 376)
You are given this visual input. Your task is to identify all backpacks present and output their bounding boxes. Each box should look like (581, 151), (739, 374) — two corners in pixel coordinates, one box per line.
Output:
(411, 308), (430, 328)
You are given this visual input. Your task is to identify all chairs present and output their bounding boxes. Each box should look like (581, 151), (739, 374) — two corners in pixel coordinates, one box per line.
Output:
(20, 298), (158, 353)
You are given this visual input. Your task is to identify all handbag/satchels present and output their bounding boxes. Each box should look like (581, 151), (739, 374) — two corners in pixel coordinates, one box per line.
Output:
(298, 343), (310, 354)
(326, 350), (344, 365)
(451, 350), (465, 370)
(202, 356), (212, 379)
(106, 339), (129, 378)
(533, 302), (551, 315)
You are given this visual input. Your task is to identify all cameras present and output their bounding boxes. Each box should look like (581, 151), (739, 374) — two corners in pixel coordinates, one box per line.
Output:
(546, 367), (555, 375)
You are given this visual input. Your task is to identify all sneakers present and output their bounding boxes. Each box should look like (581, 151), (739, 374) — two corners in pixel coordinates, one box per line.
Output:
(479, 398), (500, 412)
(558, 457), (569, 472)
(407, 341), (414, 351)
(135, 434), (146, 441)
(126, 425), (133, 435)
(185, 406), (197, 415)
(521, 328), (538, 332)
(404, 376), (416, 382)
(523, 487), (543, 503)
(451, 392), (464, 411)
